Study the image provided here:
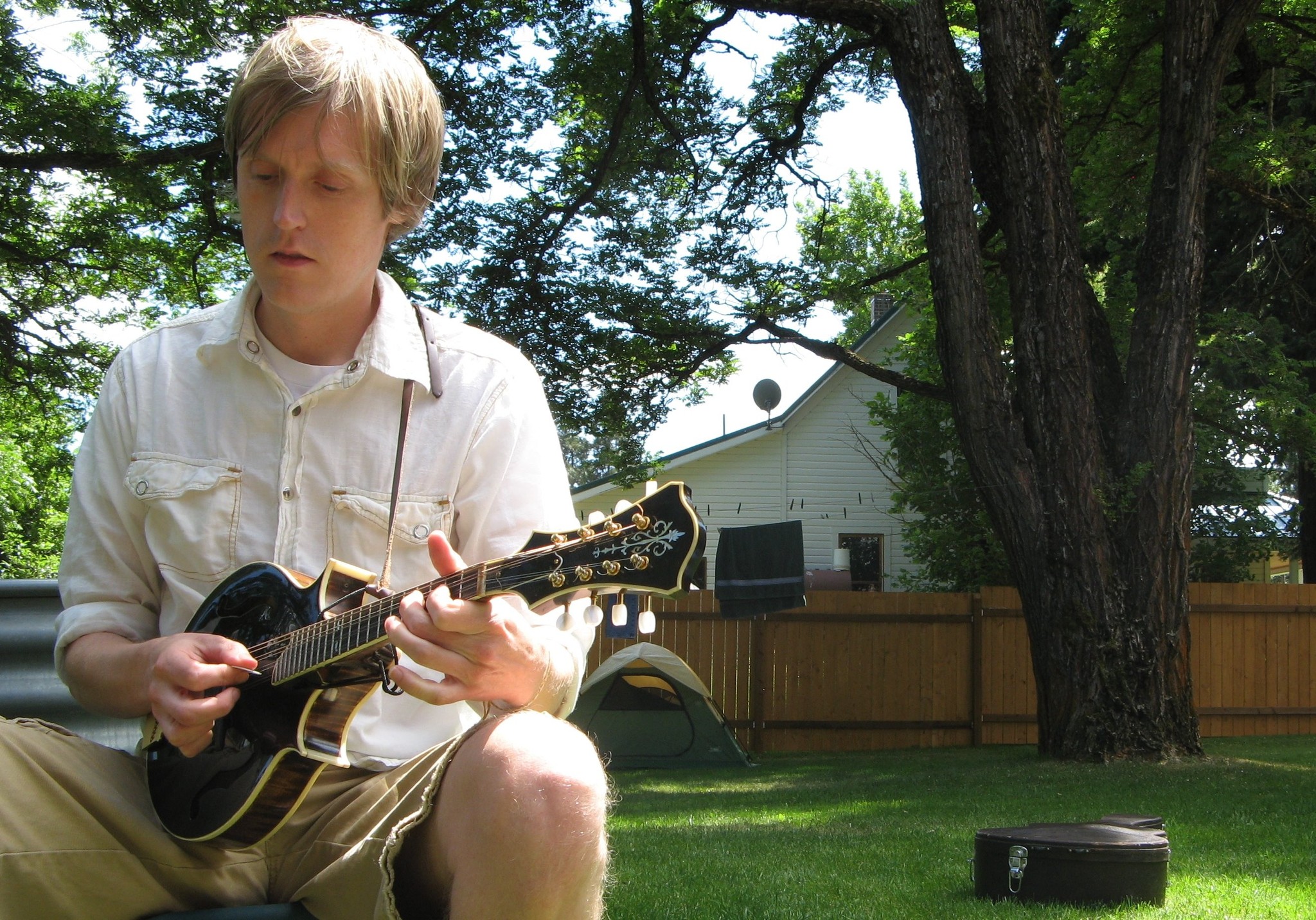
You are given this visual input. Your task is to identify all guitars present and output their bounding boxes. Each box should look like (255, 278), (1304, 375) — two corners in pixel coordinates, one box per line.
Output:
(138, 478), (707, 849)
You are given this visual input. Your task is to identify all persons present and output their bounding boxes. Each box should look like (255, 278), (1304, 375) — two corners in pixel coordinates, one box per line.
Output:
(0, 6), (612, 920)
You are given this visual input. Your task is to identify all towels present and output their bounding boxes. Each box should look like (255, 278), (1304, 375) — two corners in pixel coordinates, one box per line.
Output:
(605, 594), (638, 639)
(712, 519), (807, 622)
